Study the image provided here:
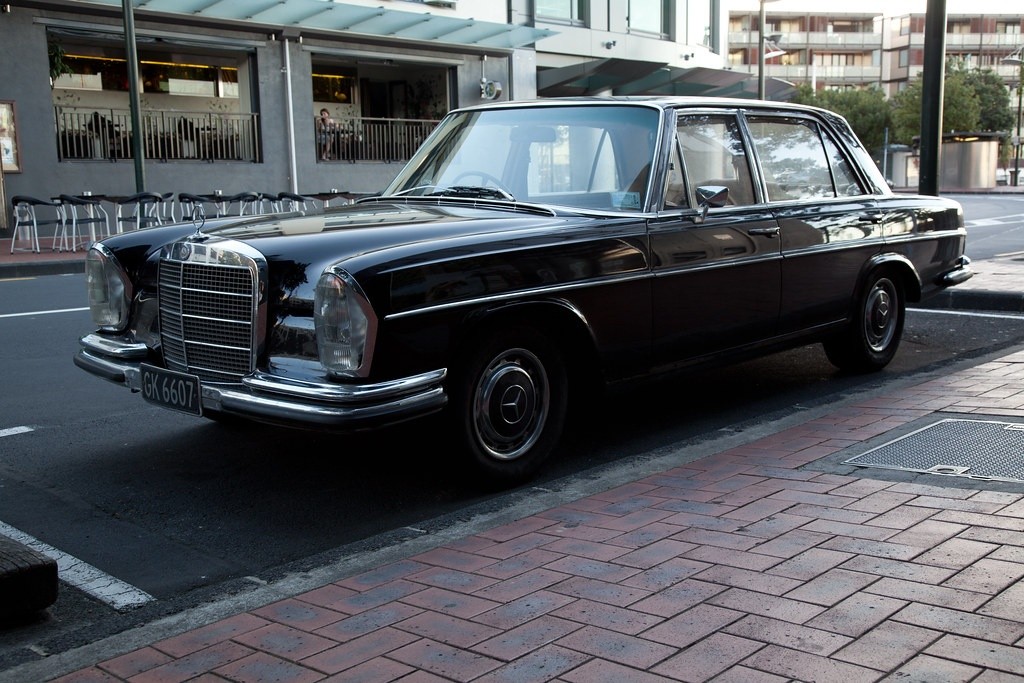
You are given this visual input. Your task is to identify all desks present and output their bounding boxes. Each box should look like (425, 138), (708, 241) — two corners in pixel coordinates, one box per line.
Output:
(84, 124), (123, 159)
(196, 194), (250, 216)
(302, 193), (340, 208)
(51, 195), (106, 251)
(196, 128), (216, 158)
(325, 131), (362, 159)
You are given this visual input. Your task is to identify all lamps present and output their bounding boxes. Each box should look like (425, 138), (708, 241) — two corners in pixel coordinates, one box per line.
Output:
(3, 4), (11, 13)
(297, 36), (302, 43)
(685, 53), (693, 60)
(607, 40), (616, 49)
(269, 33), (275, 41)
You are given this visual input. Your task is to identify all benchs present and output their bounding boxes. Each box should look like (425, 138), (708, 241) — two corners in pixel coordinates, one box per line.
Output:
(667, 177), (850, 206)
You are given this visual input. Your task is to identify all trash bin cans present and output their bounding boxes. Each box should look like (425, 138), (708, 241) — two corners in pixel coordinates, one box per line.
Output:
(1010, 170), (1020, 186)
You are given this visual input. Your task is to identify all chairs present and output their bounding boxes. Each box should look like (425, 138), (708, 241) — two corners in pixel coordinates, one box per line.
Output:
(11, 191), (380, 253)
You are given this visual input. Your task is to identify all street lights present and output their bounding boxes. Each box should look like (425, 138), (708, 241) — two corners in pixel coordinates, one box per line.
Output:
(1001, 57), (1024, 188)
(755, 0), (786, 101)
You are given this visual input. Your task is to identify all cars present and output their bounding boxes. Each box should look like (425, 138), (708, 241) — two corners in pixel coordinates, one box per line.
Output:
(72, 93), (982, 488)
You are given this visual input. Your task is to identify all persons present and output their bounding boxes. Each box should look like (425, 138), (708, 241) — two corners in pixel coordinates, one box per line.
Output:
(317, 109), (339, 160)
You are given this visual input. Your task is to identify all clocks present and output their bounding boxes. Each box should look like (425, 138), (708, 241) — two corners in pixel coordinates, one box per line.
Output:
(484, 80), (502, 100)
